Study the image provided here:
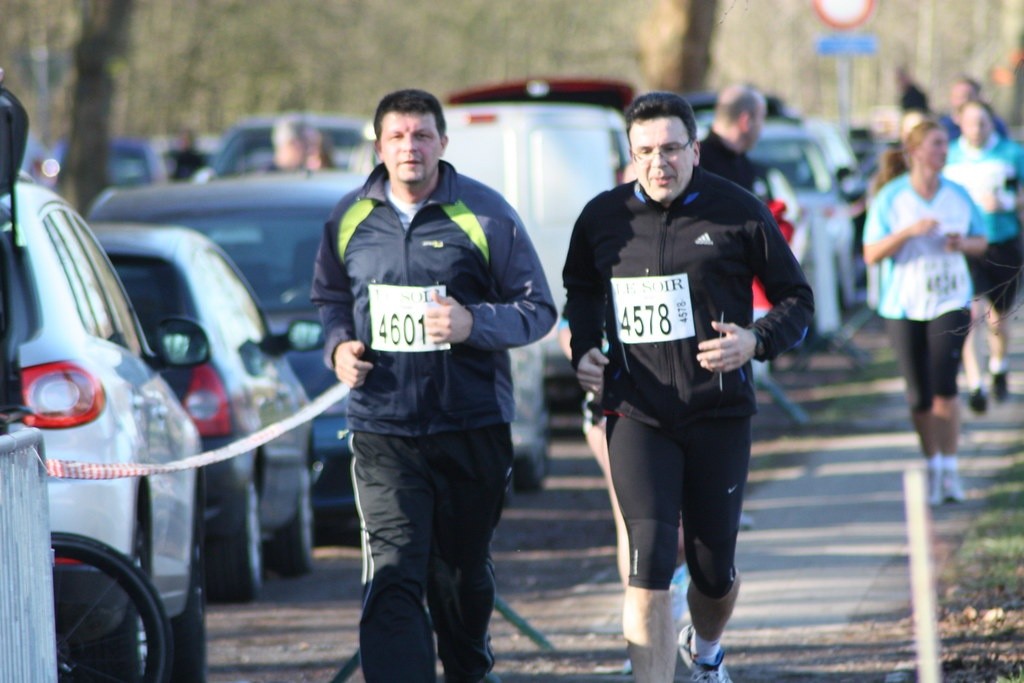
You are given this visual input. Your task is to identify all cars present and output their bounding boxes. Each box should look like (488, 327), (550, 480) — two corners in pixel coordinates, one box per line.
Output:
(21, 73), (904, 502)
(67, 171), (367, 544)
(0, 182), (214, 683)
(78, 218), (327, 607)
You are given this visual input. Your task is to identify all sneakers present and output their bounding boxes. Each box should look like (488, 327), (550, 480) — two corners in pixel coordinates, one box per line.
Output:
(678, 624), (734, 683)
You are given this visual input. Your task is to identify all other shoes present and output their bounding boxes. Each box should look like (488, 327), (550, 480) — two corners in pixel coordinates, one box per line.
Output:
(990, 373), (1008, 402)
(967, 387), (986, 413)
(926, 467), (944, 506)
(943, 470), (962, 502)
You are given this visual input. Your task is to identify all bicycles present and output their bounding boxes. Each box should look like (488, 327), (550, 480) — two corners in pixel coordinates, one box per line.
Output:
(46, 530), (179, 683)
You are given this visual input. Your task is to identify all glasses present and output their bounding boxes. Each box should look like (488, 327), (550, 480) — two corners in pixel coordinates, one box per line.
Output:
(632, 139), (691, 165)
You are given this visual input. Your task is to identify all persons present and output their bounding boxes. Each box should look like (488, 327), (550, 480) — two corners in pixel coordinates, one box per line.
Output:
(163, 127), (208, 181)
(268, 115), (333, 171)
(698, 83), (764, 192)
(562, 94), (817, 683)
(863, 68), (1023, 507)
(309, 90), (558, 682)
